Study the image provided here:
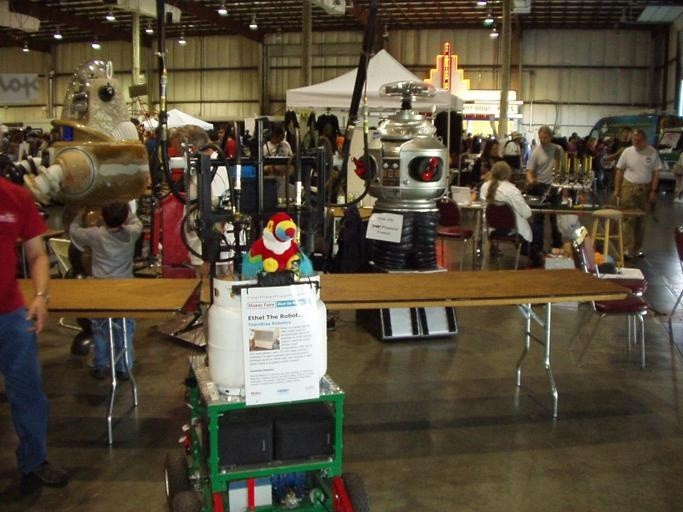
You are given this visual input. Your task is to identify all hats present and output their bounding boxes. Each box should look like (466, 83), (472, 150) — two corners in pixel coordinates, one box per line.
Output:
(510, 131), (524, 140)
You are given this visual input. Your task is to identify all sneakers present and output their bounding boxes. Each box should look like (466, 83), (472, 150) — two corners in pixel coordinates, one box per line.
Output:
(623, 247), (646, 260)
(30, 459), (71, 489)
(90, 366), (130, 381)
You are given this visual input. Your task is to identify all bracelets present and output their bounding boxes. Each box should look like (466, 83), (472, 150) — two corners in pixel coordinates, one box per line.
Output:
(34, 290), (50, 304)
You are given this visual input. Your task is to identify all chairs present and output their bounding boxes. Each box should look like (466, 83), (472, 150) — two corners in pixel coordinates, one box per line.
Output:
(578, 236), (649, 344)
(568, 246), (649, 367)
(670, 224), (682, 320)
(481, 202), (529, 269)
(435, 198), (473, 269)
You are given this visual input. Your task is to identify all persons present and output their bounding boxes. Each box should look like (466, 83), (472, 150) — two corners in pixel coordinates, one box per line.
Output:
(672, 155), (682, 206)
(127, 117), (291, 264)
(0, 168), (67, 486)
(468, 125), (663, 260)
(67, 201), (144, 380)
(0, 126), (51, 164)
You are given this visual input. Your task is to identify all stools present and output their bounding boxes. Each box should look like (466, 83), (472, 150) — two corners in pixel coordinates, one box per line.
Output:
(590, 209), (625, 268)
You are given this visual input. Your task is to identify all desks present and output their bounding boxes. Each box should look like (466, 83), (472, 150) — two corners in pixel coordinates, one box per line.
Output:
(452, 200), (644, 269)
(15, 273), (202, 446)
(196, 269), (630, 417)
